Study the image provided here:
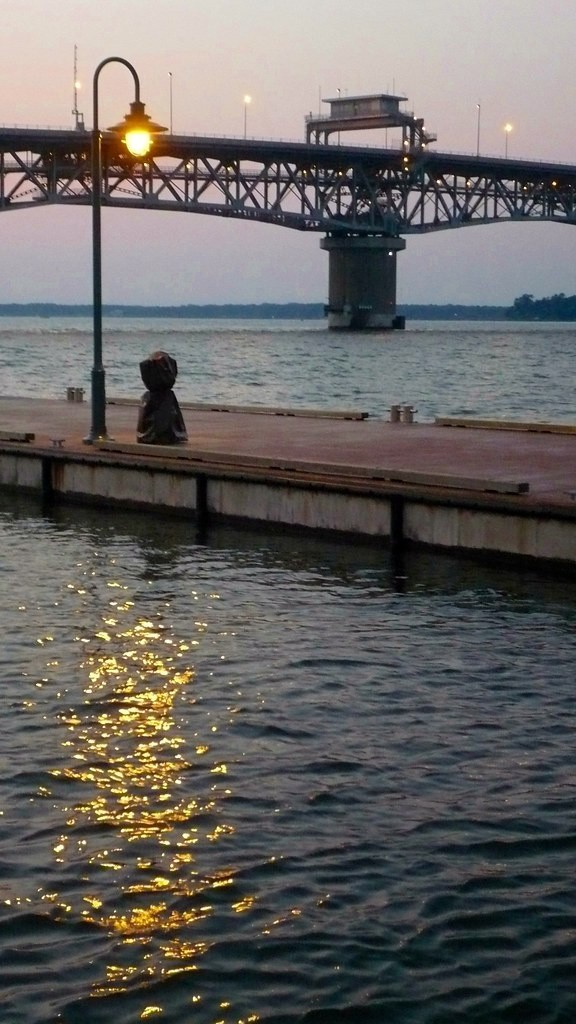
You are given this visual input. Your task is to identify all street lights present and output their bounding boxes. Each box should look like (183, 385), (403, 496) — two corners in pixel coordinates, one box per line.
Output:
(78, 53), (165, 448)
(167, 71), (173, 136)
(475, 104), (481, 156)
(243, 95), (251, 140)
(504, 124), (513, 159)
